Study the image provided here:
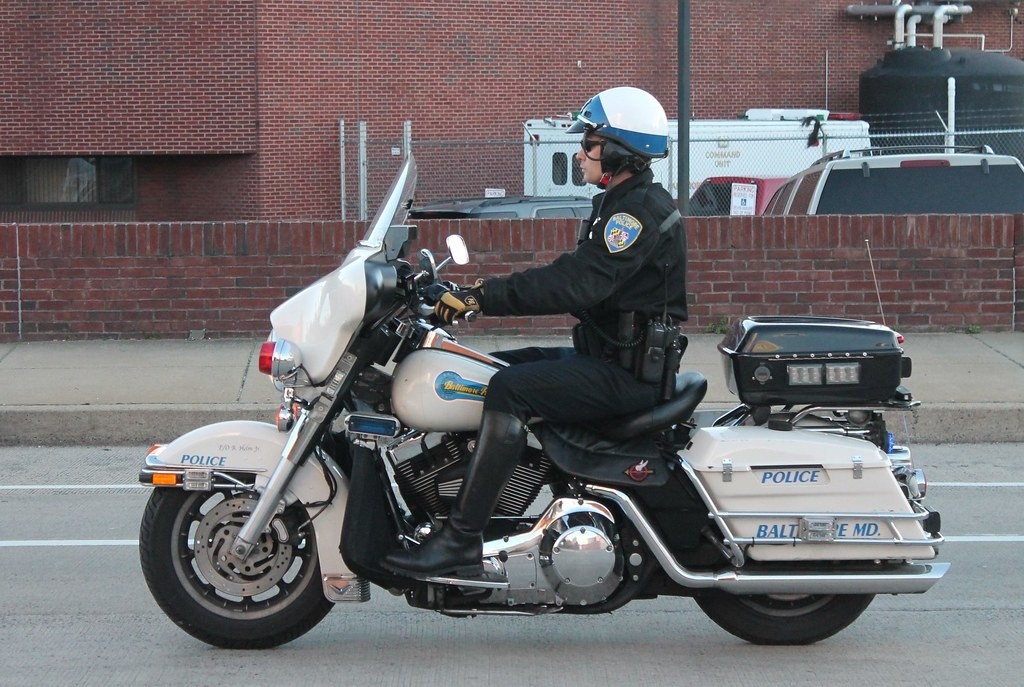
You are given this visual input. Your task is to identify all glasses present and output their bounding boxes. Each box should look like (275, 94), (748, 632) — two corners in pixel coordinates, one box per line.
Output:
(580, 136), (606, 153)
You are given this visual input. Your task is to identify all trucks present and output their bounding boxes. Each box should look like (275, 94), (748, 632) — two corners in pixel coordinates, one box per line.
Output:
(523, 108), (872, 204)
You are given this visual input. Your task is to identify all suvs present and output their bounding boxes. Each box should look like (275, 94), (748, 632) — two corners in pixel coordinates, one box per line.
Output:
(762, 146), (1024, 216)
(408, 193), (594, 221)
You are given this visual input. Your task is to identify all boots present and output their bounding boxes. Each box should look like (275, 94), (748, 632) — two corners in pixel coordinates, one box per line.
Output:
(379, 410), (530, 579)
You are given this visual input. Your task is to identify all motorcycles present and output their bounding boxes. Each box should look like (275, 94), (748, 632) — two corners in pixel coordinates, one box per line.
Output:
(135, 149), (952, 652)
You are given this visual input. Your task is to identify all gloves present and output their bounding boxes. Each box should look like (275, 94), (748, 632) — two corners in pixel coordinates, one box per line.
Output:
(435, 277), (485, 326)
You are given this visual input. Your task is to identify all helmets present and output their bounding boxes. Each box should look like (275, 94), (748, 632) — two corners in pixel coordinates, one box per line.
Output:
(565, 87), (669, 157)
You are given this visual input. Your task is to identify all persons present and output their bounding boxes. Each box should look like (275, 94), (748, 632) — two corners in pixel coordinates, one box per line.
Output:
(376, 88), (687, 578)
